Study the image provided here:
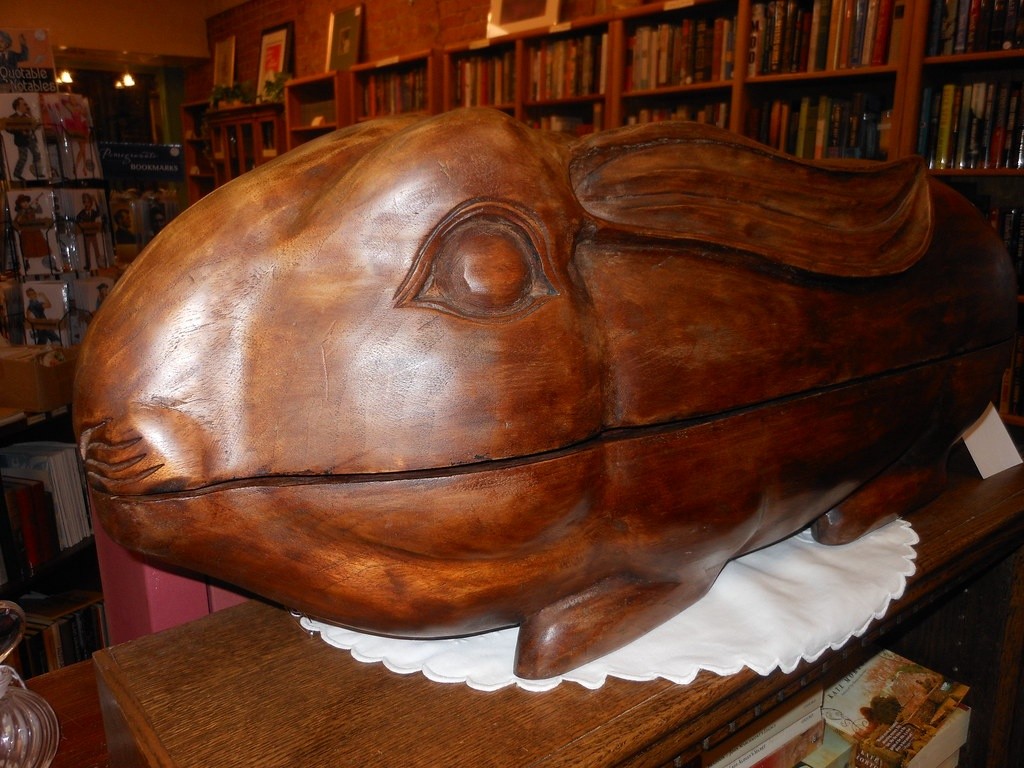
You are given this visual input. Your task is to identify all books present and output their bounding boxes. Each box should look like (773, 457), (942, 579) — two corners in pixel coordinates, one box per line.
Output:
(0, 92), (180, 681)
(709, 650), (971, 768)
(363, 0), (1024, 249)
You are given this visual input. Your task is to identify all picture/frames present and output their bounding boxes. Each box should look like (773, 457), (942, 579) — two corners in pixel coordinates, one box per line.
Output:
(485, 0), (563, 40)
(255, 20), (296, 105)
(324, 3), (364, 72)
(213, 35), (237, 96)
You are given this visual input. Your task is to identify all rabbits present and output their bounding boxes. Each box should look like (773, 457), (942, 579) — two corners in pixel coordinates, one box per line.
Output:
(72, 108), (1019, 679)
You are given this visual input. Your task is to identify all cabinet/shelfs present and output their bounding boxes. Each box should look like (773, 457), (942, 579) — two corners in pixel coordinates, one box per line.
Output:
(95, 437), (1024, 768)
(181, 0), (1024, 229)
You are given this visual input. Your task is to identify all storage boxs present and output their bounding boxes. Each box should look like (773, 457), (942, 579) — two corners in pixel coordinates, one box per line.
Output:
(0, 344), (77, 413)
